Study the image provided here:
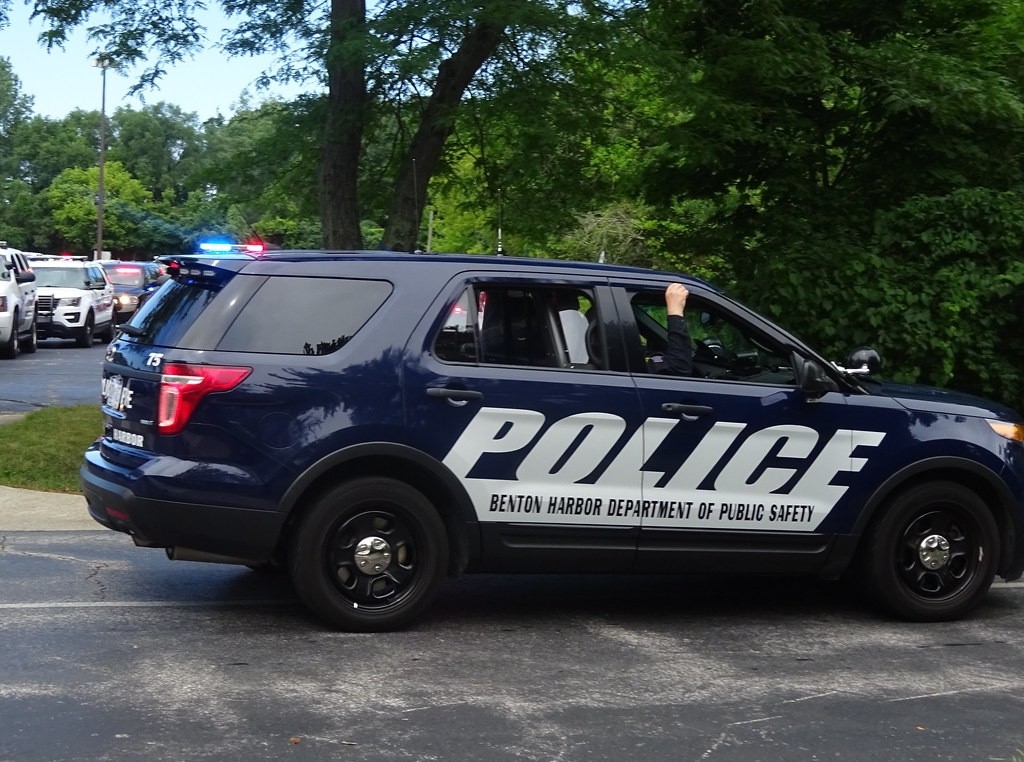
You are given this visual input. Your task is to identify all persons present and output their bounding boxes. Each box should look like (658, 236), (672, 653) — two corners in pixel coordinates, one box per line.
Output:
(635, 283), (696, 377)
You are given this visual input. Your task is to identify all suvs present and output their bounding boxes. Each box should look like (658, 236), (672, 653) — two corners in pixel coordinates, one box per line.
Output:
(26, 253), (118, 348)
(77, 233), (1024, 635)
(98, 256), (170, 326)
(0, 239), (41, 360)
